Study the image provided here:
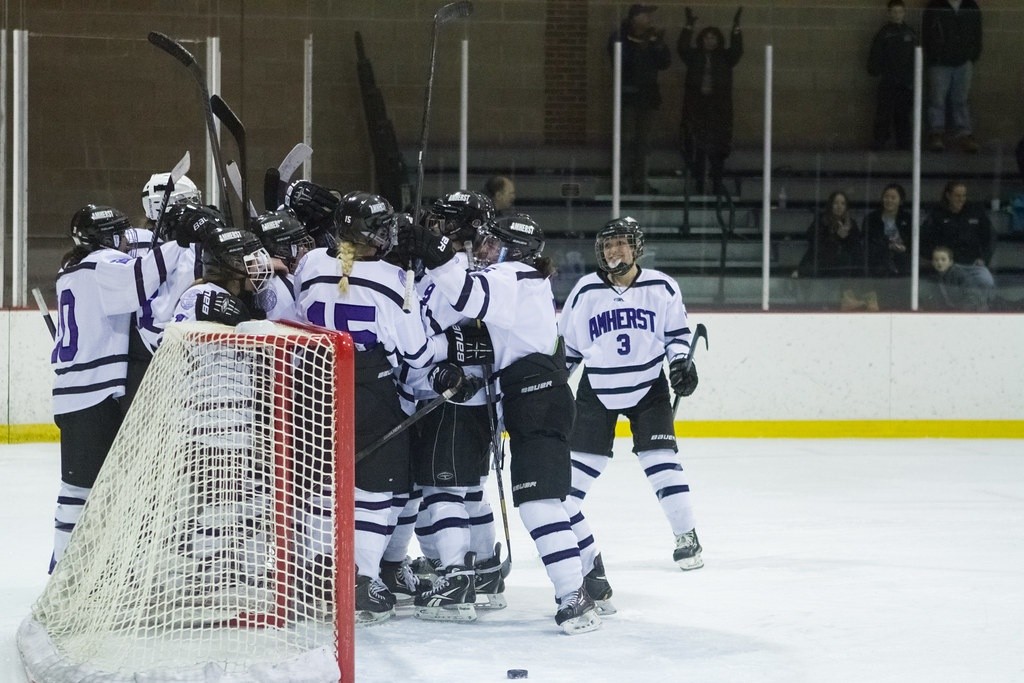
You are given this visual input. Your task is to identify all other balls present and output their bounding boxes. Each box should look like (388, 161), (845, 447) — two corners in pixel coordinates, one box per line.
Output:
(507, 669), (528, 679)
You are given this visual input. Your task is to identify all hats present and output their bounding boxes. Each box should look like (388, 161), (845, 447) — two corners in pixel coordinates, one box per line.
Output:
(628, 4), (658, 17)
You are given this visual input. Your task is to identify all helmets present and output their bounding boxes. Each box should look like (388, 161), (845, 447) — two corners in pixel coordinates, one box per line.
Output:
(335, 190), (397, 258)
(204, 227), (274, 294)
(468, 213), (546, 268)
(251, 210), (315, 274)
(141, 173), (203, 220)
(418, 190), (495, 245)
(594, 215), (645, 276)
(70, 204), (138, 261)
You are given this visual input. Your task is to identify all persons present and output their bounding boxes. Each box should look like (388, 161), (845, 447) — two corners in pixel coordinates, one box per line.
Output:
(48, 171), (617, 636)
(676, 5), (744, 193)
(560, 217), (704, 571)
(922, 0), (984, 153)
(794, 182), (998, 312)
(608, 4), (672, 193)
(866, 0), (918, 149)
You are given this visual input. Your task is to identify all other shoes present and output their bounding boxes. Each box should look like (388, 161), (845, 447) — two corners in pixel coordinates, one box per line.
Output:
(839, 289), (879, 313)
(630, 172), (727, 196)
(925, 131), (979, 153)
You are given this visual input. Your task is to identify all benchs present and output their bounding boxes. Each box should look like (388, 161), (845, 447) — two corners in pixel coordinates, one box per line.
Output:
(397, 147), (1024, 313)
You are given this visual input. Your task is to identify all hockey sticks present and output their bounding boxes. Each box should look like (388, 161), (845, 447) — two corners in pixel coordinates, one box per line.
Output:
(197, 387), (459, 574)
(208, 95), (251, 229)
(143, 30), (234, 228)
(672, 323), (709, 420)
(482, 361), (513, 580)
(412, 0), (474, 227)
(147, 151), (192, 254)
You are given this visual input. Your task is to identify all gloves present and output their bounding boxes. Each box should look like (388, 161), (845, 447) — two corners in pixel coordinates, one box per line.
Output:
(195, 291), (251, 326)
(427, 361), (480, 403)
(669, 359), (698, 397)
(443, 323), (494, 365)
(175, 203), (227, 246)
(397, 224), (457, 270)
(733, 6), (743, 27)
(685, 7), (699, 26)
(285, 180), (339, 226)
(389, 213), (413, 246)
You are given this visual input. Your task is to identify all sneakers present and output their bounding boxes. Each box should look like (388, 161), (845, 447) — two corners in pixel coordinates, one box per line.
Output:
(349, 542), (508, 625)
(554, 586), (603, 635)
(582, 553), (618, 615)
(673, 528), (704, 570)
